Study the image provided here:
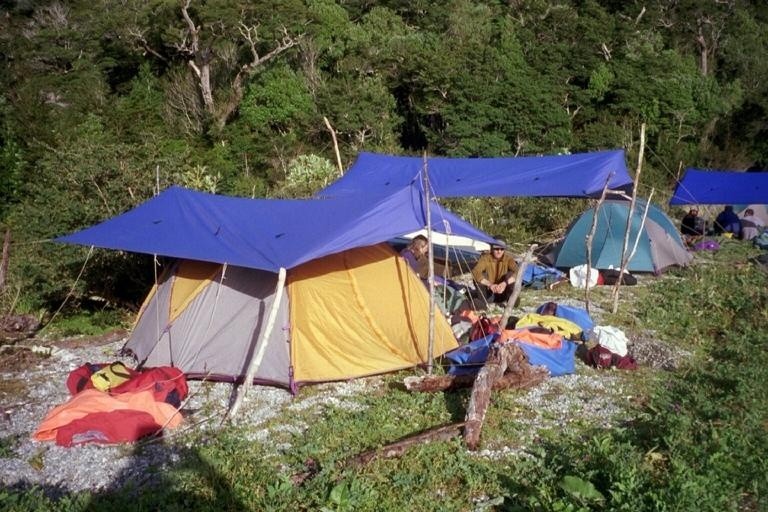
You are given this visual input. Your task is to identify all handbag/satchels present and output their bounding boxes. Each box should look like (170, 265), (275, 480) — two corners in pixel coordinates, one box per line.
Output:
(470, 320), (489, 340)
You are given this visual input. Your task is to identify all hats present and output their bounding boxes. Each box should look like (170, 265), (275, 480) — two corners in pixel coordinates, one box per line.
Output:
(491, 235), (507, 249)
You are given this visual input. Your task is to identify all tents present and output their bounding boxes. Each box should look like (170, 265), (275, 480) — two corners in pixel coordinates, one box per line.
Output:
(545, 196), (693, 277)
(121, 242), (461, 396)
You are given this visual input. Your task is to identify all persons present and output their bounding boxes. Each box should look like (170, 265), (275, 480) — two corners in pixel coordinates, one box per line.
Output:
(399, 235), (429, 275)
(739, 209), (764, 240)
(713, 206), (742, 239)
(681, 207), (708, 235)
(472, 239), (520, 307)
